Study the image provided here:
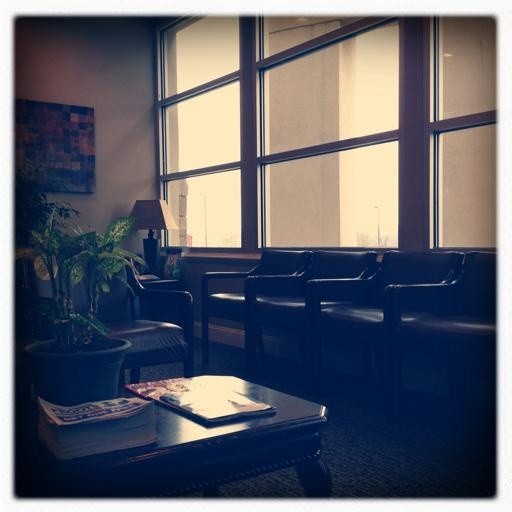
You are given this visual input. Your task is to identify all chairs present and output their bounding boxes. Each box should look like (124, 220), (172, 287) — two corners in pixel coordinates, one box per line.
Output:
(16, 266), (194, 383)
(200, 248), (496, 423)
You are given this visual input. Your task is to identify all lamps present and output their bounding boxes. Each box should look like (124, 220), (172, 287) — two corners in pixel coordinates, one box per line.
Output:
(124, 199), (180, 270)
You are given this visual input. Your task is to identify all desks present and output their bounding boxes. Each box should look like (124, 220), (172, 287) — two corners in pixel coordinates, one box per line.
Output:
(26, 374), (333, 498)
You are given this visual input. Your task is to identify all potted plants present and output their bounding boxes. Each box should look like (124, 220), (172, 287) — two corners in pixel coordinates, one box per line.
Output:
(15, 191), (151, 405)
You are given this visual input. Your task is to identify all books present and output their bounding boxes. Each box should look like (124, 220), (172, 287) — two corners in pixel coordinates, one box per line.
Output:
(37, 375), (275, 462)
